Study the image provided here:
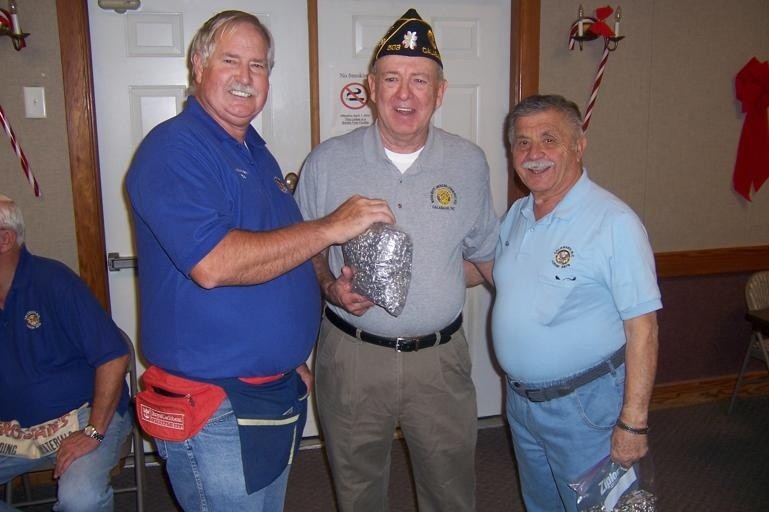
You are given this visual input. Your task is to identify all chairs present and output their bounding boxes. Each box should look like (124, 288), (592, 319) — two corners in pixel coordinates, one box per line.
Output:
(6, 328), (147, 512)
(727, 271), (769, 414)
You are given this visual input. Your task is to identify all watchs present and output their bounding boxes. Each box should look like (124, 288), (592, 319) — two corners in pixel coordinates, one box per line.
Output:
(82, 424), (106, 443)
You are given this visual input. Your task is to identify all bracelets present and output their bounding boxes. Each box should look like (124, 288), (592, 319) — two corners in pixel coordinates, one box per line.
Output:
(613, 417), (653, 435)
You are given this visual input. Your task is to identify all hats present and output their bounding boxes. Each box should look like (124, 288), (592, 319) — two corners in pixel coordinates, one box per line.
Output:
(372, 8), (443, 65)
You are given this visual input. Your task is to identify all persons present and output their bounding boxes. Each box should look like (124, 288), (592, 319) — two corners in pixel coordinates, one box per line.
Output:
(123, 11), (396, 512)
(462, 95), (664, 512)
(290, 11), (502, 512)
(0, 194), (137, 511)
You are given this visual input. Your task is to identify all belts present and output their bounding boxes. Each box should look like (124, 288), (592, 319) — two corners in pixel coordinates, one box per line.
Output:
(325, 306), (462, 352)
(504, 346), (626, 402)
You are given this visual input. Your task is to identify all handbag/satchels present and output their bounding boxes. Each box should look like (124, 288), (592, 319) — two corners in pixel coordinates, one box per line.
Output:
(135, 365), (228, 440)
